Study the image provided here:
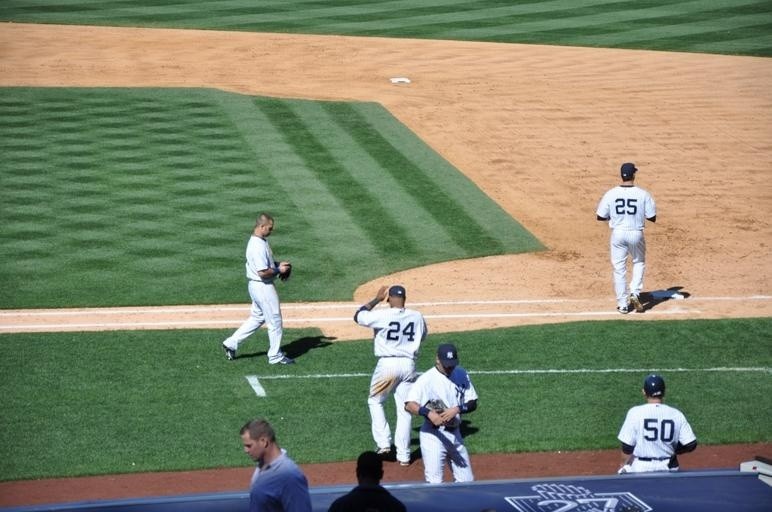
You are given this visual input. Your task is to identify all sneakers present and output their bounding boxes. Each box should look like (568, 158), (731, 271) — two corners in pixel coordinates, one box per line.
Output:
(399, 461), (409, 466)
(269, 357), (295, 364)
(617, 306), (628, 313)
(375, 448), (390, 454)
(223, 344), (236, 360)
(630, 293), (643, 312)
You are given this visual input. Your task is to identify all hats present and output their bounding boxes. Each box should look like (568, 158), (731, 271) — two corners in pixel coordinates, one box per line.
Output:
(438, 344), (457, 367)
(386, 286), (405, 303)
(621, 163), (637, 176)
(644, 375), (665, 396)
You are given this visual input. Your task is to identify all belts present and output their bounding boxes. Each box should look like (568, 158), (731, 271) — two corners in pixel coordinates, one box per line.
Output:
(638, 457), (671, 461)
(434, 423), (461, 432)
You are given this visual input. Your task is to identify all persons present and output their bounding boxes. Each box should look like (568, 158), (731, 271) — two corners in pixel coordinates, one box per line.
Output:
(354, 284), (427, 463)
(240, 420), (311, 512)
(597, 162), (656, 314)
(402, 345), (480, 483)
(617, 376), (696, 474)
(325, 450), (409, 510)
(221, 212), (298, 366)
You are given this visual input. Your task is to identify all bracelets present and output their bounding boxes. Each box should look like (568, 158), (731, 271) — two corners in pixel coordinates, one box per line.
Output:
(458, 404), (468, 414)
(418, 406), (430, 416)
(369, 298), (378, 308)
(274, 262), (280, 267)
(274, 267), (280, 274)
